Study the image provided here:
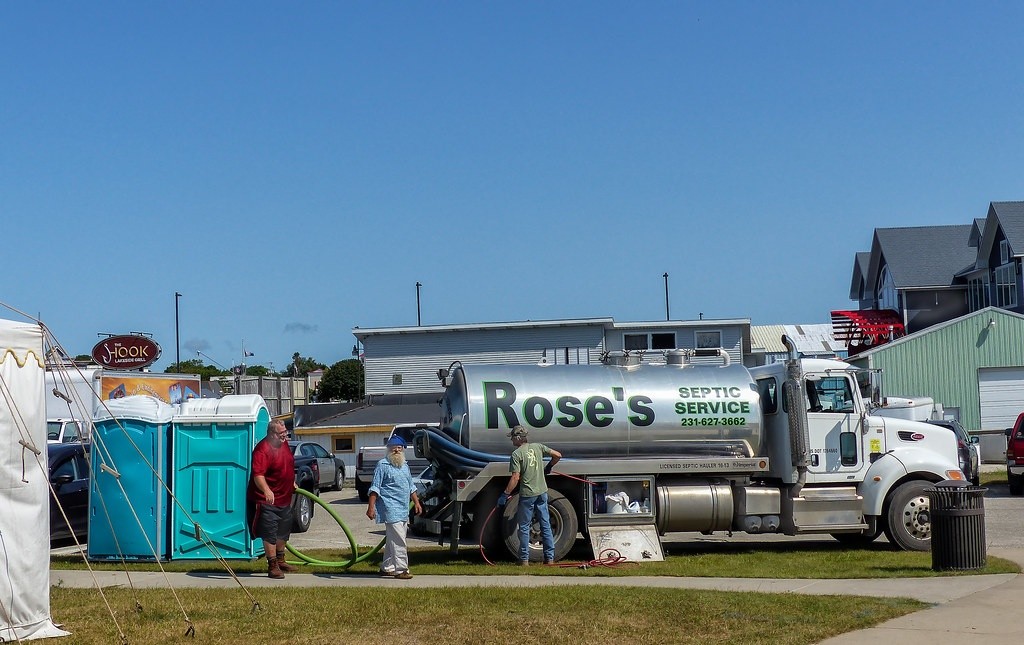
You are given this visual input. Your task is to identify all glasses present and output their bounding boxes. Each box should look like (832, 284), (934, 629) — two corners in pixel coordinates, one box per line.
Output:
(274, 429), (288, 435)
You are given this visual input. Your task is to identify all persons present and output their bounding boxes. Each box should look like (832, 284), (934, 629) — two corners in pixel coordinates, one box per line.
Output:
(496, 425), (561, 566)
(365, 436), (423, 580)
(252, 418), (300, 579)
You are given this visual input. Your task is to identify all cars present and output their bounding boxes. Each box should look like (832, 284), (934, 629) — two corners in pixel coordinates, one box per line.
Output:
(412, 463), (434, 492)
(286, 440), (345, 491)
(46, 443), (90, 540)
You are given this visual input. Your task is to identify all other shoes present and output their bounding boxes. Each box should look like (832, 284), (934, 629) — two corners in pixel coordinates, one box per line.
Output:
(543, 560), (553, 564)
(395, 570), (413, 579)
(515, 561), (529, 567)
(380, 570), (395, 576)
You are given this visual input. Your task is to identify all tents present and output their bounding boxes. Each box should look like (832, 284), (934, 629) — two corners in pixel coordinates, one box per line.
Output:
(0, 302), (261, 645)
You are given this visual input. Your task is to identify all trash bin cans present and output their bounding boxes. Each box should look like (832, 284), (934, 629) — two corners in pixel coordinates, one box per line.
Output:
(923, 480), (989, 570)
(973, 443), (980, 466)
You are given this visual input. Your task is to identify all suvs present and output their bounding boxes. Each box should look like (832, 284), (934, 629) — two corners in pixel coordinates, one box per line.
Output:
(1004, 412), (1024, 496)
(915, 418), (979, 487)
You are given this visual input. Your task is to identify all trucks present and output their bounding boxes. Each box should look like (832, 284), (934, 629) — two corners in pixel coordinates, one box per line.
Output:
(46, 418), (89, 444)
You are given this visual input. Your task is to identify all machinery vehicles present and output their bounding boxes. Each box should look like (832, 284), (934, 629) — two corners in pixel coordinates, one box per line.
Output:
(414, 336), (967, 564)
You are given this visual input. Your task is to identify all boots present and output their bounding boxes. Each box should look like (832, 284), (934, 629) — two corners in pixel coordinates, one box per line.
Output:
(276, 555), (299, 571)
(267, 556), (284, 579)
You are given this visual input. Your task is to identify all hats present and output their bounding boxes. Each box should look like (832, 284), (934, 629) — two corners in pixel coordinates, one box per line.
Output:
(506, 425), (527, 437)
(386, 434), (407, 449)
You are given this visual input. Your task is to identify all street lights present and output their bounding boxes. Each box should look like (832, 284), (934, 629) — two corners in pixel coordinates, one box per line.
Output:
(416, 282), (422, 325)
(662, 273), (669, 321)
(175, 292), (183, 373)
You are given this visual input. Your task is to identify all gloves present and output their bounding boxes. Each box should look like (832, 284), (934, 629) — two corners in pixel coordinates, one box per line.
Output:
(496, 490), (511, 507)
(544, 462), (553, 474)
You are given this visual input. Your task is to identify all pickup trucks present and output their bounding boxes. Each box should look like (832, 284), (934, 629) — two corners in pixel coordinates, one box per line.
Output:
(291, 455), (319, 533)
(355, 423), (440, 502)
